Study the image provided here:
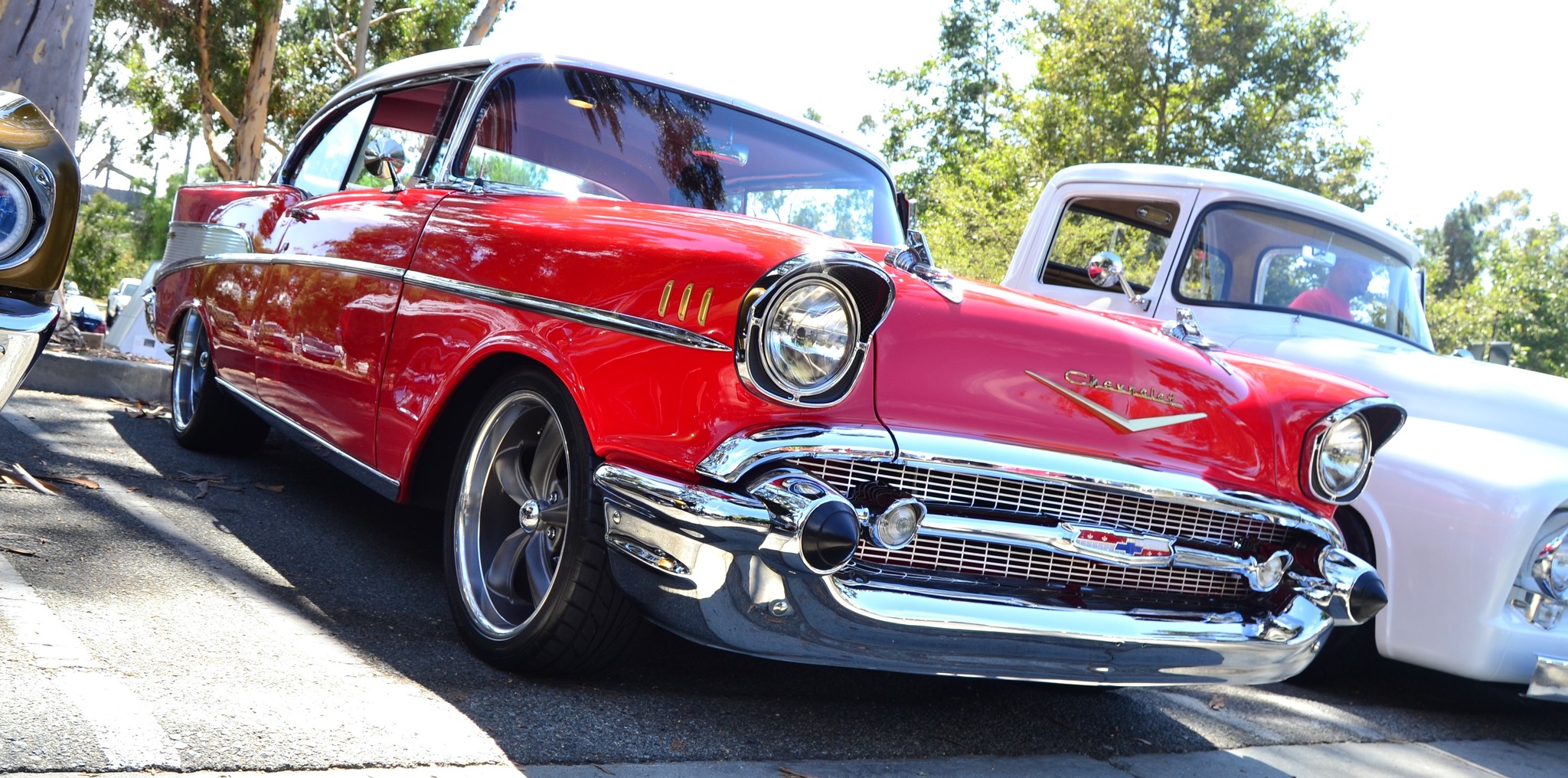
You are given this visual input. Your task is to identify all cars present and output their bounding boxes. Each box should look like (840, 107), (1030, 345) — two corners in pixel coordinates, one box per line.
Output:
(0, 1), (97, 412)
(63, 292), (107, 339)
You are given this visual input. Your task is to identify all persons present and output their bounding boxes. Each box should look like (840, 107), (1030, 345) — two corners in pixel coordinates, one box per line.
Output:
(1288, 257), (1373, 321)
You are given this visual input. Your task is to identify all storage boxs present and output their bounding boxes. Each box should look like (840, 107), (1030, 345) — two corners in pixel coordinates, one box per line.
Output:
(80, 332), (105, 349)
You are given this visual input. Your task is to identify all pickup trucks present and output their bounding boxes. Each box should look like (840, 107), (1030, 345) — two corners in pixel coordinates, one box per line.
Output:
(999, 164), (1568, 698)
(139, 45), (1408, 692)
(107, 277), (142, 327)
